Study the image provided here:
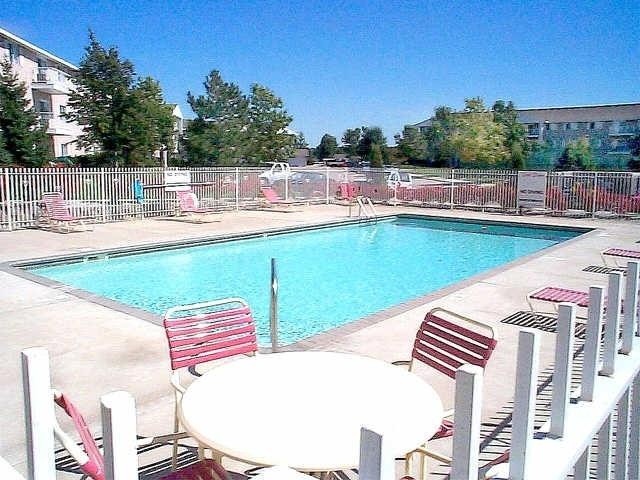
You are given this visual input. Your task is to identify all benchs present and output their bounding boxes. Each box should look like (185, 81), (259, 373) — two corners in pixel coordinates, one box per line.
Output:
(525, 284), (627, 329)
(600, 246), (640, 269)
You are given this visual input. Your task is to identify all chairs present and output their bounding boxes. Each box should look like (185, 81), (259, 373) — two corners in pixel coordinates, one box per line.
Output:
(36, 192), (93, 234)
(258, 186), (296, 213)
(175, 189), (222, 224)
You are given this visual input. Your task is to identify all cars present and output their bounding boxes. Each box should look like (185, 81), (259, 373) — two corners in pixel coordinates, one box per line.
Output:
(253, 157), (412, 205)
